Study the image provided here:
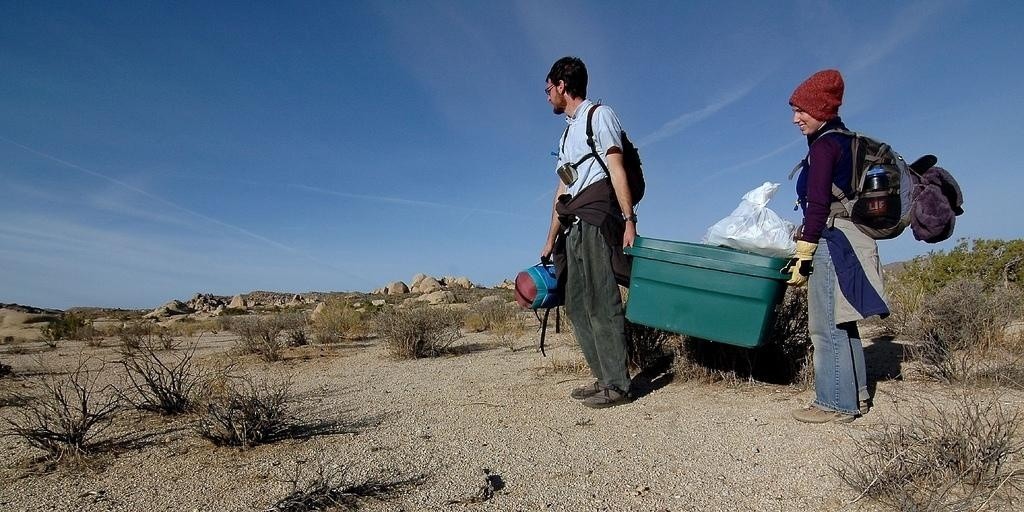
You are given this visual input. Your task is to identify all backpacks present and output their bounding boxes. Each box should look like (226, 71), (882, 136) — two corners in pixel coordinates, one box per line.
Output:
(562, 103), (645, 207)
(806, 129), (922, 242)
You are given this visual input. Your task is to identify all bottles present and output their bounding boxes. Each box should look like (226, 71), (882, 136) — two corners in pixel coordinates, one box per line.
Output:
(865, 167), (889, 192)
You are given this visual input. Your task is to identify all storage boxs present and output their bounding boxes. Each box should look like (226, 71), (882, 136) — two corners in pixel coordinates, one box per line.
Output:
(622, 236), (800, 351)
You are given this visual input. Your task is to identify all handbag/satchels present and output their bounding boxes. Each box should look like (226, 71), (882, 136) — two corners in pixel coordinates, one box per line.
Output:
(514, 255), (565, 310)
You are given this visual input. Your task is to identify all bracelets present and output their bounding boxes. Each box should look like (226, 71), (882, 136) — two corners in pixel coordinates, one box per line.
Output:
(622, 214), (639, 224)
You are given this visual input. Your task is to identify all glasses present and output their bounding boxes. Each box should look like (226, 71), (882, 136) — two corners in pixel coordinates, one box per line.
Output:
(544, 84), (556, 94)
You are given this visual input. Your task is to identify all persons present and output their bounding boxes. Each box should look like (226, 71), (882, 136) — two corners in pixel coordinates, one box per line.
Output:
(778, 68), (873, 424)
(540, 56), (638, 408)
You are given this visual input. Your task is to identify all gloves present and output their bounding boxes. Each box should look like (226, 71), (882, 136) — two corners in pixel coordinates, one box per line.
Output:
(780, 240), (818, 286)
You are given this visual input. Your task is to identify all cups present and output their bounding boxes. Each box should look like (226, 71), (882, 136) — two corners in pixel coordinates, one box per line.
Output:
(557, 163), (579, 187)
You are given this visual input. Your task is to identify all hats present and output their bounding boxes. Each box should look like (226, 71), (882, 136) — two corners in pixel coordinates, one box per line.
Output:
(789, 70), (845, 121)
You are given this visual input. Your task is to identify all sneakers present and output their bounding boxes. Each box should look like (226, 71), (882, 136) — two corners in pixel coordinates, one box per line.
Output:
(791, 400), (868, 424)
(571, 382), (633, 409)
